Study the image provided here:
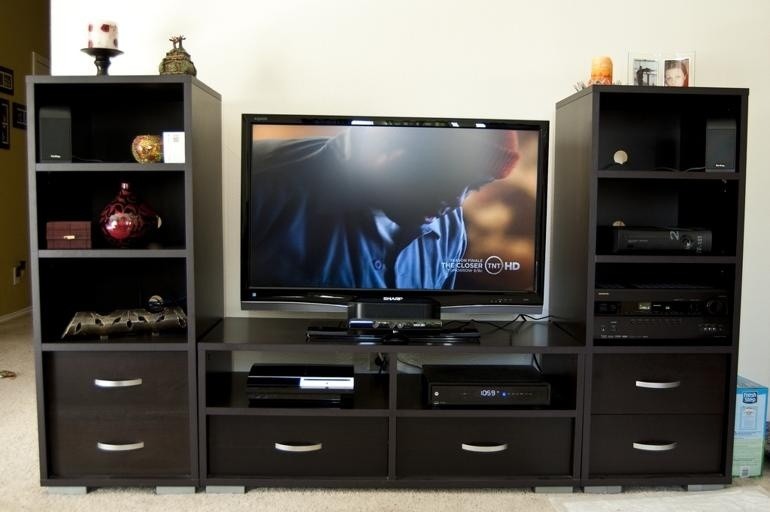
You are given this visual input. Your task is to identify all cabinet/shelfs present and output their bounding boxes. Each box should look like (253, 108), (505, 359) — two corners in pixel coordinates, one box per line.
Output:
(551, 85), (750, 354)
(25, 72), (224, 341)
(582, 352), (737, 493)
(32, 340), (198, 494)
(199, 316), (582, 495)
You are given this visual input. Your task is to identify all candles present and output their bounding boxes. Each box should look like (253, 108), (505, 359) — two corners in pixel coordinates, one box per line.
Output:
(591, 55), (613, 86)
(88, 20), (117, 49)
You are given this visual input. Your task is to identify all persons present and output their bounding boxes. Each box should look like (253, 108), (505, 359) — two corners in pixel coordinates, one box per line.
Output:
(250, 126), (521, 290)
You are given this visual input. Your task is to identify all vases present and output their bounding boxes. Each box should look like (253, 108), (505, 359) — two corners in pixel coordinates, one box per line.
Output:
(96, 182), (158, 249)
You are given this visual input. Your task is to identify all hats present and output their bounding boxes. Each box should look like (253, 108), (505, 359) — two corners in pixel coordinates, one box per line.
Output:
(436, 129), (520, 180)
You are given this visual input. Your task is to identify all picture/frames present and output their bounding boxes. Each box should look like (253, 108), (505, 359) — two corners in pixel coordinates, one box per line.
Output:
(661, 50), (695, 87)
(0, 65), (14, 96)
(0, 97), (11, 150)
(11, 101), (26, 129)
(628, 51), (660, 85)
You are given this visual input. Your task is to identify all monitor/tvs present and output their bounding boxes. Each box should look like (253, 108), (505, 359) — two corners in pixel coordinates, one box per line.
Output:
(240, 114), (549, 337)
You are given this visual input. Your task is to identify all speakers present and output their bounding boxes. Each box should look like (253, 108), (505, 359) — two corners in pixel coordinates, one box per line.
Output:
(704, 120), (737, 173)
(39, 108), (72, 162)
